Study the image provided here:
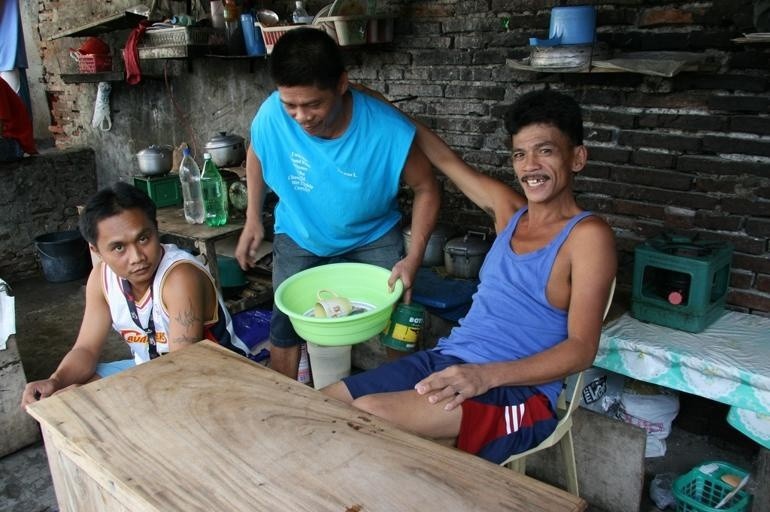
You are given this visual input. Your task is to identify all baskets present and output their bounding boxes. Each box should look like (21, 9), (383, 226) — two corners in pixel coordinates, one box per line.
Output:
(254, 22), (320, 55)
(77, 54), (113, 74)
(145, 26), (212, 47)
(672, 460), (753, 512)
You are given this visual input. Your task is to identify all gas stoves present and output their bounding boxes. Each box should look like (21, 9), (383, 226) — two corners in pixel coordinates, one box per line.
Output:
(133, 172), (182, 209)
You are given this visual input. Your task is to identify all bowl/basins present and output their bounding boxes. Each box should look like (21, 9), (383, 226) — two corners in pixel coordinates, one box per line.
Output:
(273, 262), (404, 347)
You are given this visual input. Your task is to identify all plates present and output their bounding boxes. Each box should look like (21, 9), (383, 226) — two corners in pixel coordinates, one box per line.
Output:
(311, 0), (368, 25)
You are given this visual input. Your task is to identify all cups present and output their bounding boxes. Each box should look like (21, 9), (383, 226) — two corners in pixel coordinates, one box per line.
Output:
(313, 289), (352, 318)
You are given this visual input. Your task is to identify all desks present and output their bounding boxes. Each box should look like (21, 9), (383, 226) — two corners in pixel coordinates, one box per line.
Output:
(598, 298), (769, 451)
(23, 336), (589, 512)
(75, 199), (274, 319)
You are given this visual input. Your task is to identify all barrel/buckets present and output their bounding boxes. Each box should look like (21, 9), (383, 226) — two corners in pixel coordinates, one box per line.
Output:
(32, 230), (90, 283)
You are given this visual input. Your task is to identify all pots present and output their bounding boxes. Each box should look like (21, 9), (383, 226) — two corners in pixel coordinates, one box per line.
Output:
(205, 131), (246, 169)
(135, 144), (174, 176)
(442, 229), (494, 279)
(403, 223), (449, 268)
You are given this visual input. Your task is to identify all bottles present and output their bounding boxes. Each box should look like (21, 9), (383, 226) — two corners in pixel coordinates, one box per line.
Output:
(292, 0), (309, 25)
(200, 152), (227, 227)
(178, 148), (206, 225)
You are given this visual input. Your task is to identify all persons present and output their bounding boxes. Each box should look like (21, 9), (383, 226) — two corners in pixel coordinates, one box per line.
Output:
(19, 180), (251, 413)
(314, 84), (620, 464)
(232, 24), (445, 378)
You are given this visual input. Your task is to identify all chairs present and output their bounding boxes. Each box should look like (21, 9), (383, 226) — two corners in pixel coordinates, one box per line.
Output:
(500, 274), (622, 497)
